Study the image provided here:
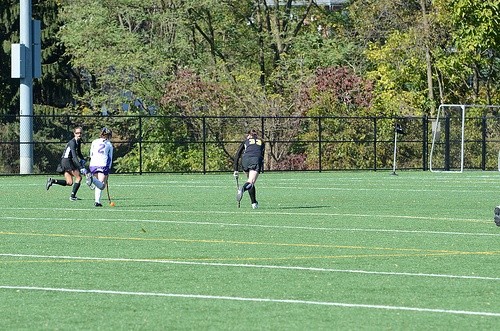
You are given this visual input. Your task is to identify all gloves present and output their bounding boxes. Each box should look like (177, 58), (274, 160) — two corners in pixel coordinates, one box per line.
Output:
(233, 171), (239, 179)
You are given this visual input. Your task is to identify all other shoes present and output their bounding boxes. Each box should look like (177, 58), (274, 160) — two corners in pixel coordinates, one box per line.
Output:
(69, 197), (81, 201)
(86, 173), (92, 186)
(251, 202), (258, 209)
(94, 202), (102, 206)
(46, 177), (52, 190)
(236, 186), (243, 201)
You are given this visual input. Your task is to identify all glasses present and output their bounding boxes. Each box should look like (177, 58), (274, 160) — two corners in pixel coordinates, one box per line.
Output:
(75, 132), (82, 134)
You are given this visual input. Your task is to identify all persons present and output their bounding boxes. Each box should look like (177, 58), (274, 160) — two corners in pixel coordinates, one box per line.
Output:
(232, 129), (265, 209)
(45, 125), (86, 201)
(85, 127), (113, 207)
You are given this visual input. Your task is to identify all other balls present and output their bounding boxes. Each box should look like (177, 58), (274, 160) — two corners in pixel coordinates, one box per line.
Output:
(110, 202), (115, 207)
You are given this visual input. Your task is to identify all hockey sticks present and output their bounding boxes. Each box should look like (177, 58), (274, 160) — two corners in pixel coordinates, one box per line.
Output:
(85, 172), (96, 191)
(236, 178), (240, 208)
(107, 177), (115, 206)
